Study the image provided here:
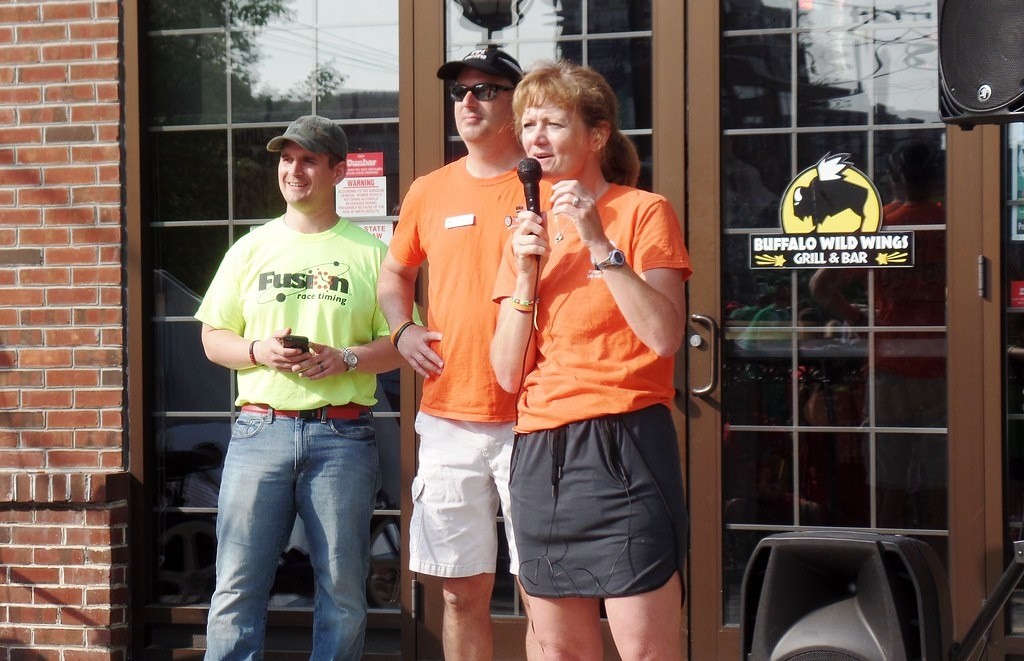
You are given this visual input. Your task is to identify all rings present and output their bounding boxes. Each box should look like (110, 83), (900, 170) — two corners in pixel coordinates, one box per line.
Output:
(573, 197), (580, 206)
(318, 363), (323, 371)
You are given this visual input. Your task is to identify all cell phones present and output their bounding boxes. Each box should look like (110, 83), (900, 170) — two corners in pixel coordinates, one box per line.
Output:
(281, 335), (309, 354)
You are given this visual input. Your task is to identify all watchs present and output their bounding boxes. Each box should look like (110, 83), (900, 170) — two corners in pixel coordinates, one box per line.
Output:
(593, 249), (626, 270)
(340, 347), (359, 372)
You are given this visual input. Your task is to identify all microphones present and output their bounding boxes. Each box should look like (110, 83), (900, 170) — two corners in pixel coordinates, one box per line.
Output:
(517, 157), (544, 263)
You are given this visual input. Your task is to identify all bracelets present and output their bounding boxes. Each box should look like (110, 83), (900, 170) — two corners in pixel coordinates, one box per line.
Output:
(249, 340), (264, 367)
(510, 296), (539, 312)
(391, 319), (415, 348)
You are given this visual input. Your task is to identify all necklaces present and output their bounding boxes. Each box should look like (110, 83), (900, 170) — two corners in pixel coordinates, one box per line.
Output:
(556, 214), (572, 241)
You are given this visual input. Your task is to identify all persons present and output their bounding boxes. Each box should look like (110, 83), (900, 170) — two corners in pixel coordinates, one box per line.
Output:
(809, 135), (947, 432)
(376, 48), (552, 661)
(490, 65), (693, 661)
(739, 282), (800, 350)
(193, 115), (425, 661)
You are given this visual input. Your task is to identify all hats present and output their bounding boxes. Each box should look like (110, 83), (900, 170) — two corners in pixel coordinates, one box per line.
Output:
(266, 114), (349, 161)
(437, 48), (523, 84)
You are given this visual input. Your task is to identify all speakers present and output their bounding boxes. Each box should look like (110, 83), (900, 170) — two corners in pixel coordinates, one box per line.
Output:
(937, 0), (1024, 131)
(740, 530), (955, 661)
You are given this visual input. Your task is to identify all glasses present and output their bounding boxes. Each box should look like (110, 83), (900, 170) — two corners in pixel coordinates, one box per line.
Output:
(447, 83), (514, 102)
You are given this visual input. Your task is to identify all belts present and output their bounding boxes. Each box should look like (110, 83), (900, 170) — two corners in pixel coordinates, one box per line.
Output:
(241, 401), (370, 421)
